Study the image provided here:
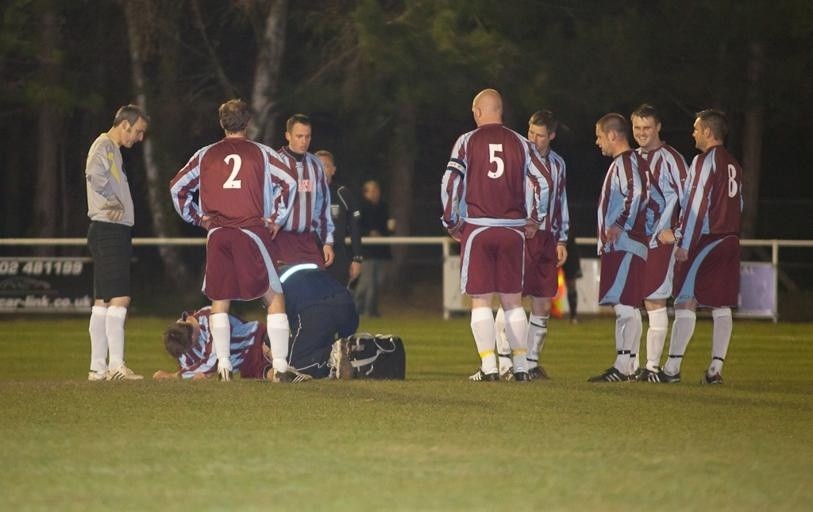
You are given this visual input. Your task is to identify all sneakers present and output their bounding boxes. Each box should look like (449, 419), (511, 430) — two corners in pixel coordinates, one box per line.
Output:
(588, 365), (683, 384)
(328, 338), (354, 380)
(217, 367), (233, 381)
(272, 366), (312, 383)
(88, 360), (143, 380)
(469, 363), (549, 382)
(699, 368), (724, 386)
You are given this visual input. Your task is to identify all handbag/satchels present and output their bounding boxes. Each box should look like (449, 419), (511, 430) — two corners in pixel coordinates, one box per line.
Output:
(346, 332), (405, 380)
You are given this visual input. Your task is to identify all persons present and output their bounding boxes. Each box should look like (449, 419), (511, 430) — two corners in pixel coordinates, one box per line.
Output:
(256, 259), (359, 381)
(620, 104), (692, 383)
(345, 179), (399, 318)
(657, 109), (745, 384)
(311, 150), (364, 292)
(266, 112), (336, 273)
(151, 304), (405, 381)
(83, 103), (151, 381)
(585, 111), (653, 382)
(169, 96), (314, 383)
(561, 231), (584, 326)
(495, 108), (569, 382)
(440, 88), (554, 383)
